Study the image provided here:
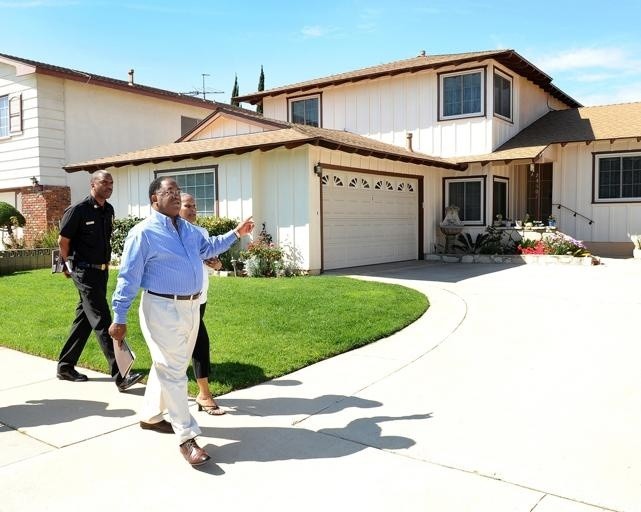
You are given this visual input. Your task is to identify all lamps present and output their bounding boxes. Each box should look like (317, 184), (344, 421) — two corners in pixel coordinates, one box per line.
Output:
(30, 176), (38, 185)
(314, 161), (322, 177)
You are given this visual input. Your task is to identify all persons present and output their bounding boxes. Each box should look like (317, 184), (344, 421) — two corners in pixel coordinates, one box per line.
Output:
(179, 192), (225, 414)
(108, 176), (256, 467)
(55, 170), (144, 393)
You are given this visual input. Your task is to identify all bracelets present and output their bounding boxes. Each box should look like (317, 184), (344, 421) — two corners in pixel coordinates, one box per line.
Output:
(233, 229), (242, 238)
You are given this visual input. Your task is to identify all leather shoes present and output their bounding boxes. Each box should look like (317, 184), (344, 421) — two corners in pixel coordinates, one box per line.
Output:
(118, 372), (145, 391)
(139, 419), (174, 433)
(179, 438), (210, 467)
(57, 370), (88, 382)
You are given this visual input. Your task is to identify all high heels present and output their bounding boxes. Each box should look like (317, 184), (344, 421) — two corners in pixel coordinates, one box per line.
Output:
(197, 399), (224, 415)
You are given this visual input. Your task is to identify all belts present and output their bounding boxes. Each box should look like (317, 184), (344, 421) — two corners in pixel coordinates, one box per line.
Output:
(79, 263), (107, 271)
(145, 290), (203, 300)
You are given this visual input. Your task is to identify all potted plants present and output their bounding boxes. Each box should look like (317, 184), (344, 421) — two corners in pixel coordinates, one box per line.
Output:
(493, 214), (557, 227)
(627, 232), (641, 259)
